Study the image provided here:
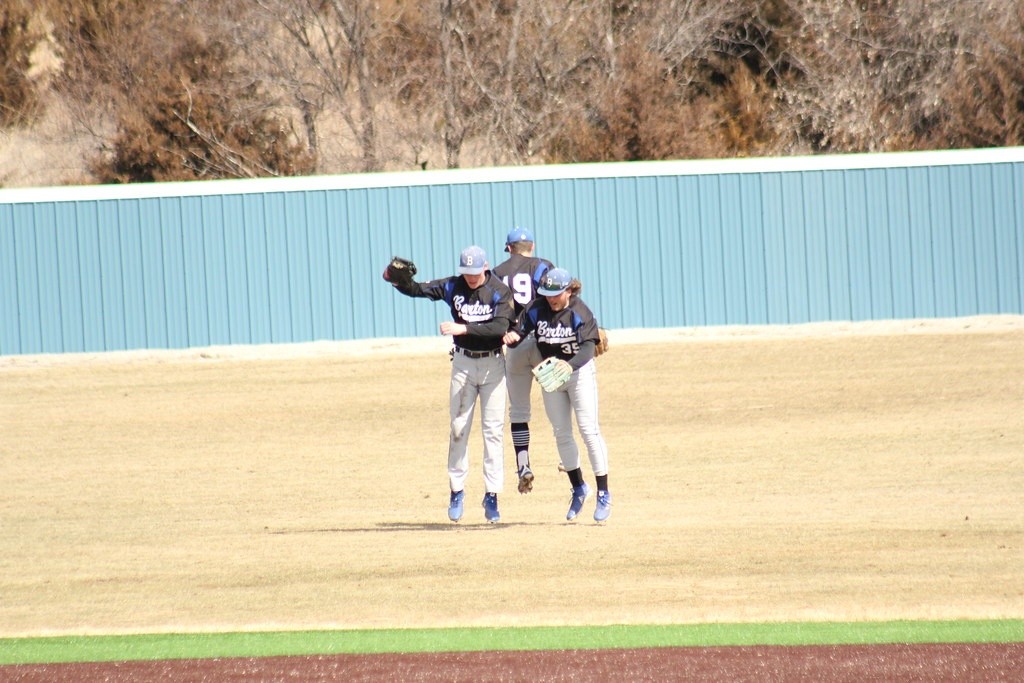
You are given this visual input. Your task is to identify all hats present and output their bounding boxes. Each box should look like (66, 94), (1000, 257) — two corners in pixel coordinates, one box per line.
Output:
(459, 245), (486, 274)
(503, 226), (534, 252)
(537, 267), (572, 296)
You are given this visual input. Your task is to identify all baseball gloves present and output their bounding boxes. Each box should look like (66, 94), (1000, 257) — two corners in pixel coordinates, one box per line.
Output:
(382, 256), (417, 289)
(531, 355), (574, 392)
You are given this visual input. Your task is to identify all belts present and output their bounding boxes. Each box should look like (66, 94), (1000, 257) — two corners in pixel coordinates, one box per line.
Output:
(454, 346), (503, 359)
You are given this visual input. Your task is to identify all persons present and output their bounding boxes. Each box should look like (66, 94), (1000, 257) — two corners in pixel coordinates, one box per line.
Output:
(386, 244), (516, 524)
(488, 226), (573, 493)
(503, 268), (612, 521)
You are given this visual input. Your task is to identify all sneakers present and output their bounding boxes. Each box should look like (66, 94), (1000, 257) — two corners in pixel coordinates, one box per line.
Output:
(558, 460), (567, 472)
(566, 483), (611, 522)
(514, 466), (535, 495)
(448, 490), (500, 522)
